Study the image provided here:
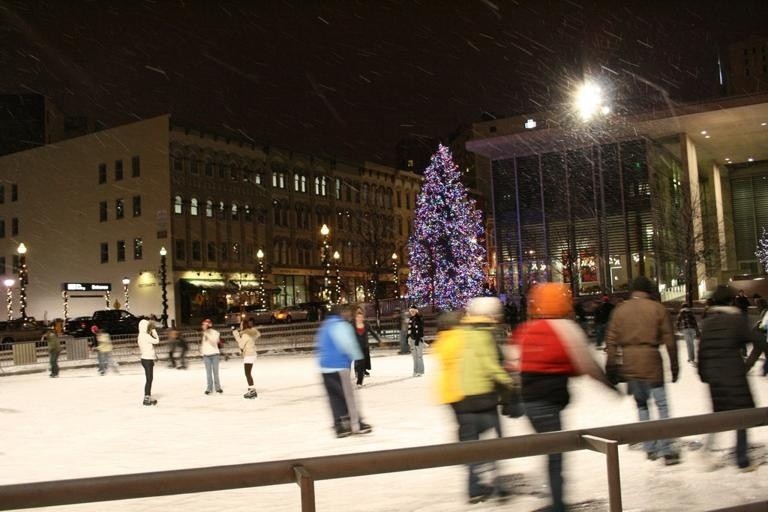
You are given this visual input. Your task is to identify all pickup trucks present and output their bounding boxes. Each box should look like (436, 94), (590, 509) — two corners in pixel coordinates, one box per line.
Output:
(61, 308), (146, 347)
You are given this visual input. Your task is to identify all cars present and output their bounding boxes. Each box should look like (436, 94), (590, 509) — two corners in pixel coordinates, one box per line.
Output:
(223, 301), (274, 327)
(0, 318), (57, 343)
(273, 305), (310, 323)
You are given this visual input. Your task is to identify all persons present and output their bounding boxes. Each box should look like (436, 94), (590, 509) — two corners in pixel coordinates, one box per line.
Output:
(698, 286), (766, 469)
(500, 281), (606, 512)
(349, 309), (383, 387)
(341, 306), (353, 322)
(432, 311), (515, 503)
(407, 306), (424, 377)
(605, 275), (681, 466)
(501, 290), (768, 377)
(314, 312), (371, 438)
(46, 318), (261, 406)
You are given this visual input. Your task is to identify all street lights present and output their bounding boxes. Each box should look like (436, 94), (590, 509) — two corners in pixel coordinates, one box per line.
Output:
(105, 290), (111, 309)
(160, 245), (168, 328)
(320, 223), (331, 313)
(333, 251), (342, 304)
(255, 249), (269, 315)
(60, 290), (70, 336)
(5, 277), (14, 320)
(391, 251), (402, 300)
(17, 242), (29, 320)
(122, 278), (130, 312)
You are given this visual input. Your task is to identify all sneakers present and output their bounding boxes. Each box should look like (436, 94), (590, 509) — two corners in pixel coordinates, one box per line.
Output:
(337, 425), (351, 437)
(665, 454), (679, 464)
(205, 391), (209, 394)
(358, 423), (371, 434)
(217, 390), (223, 393)
(244, 392), (255, 398)
